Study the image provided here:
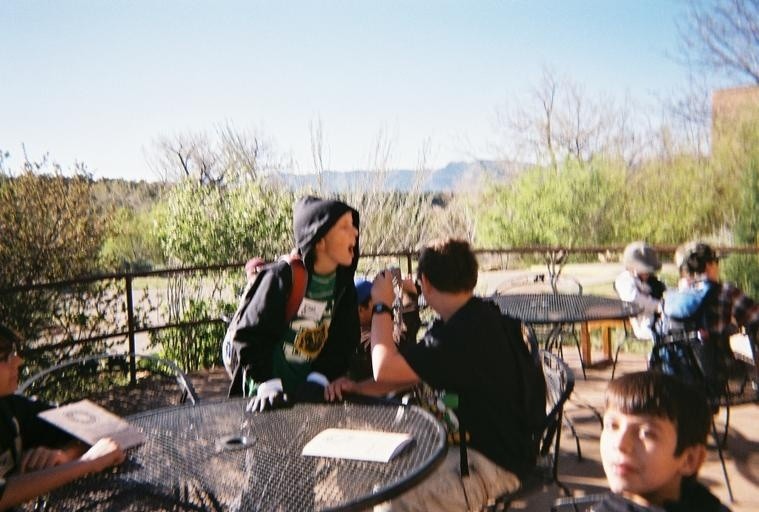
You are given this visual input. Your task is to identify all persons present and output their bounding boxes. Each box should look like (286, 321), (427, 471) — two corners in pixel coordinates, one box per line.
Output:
(324, 240), (547, 511)
(229, 194), (360, 414)
(615, 240), (666, 339)
(648, 241), (759, 414)
(581, 371), (731, 512)
(353, 274), (418, 377)
(0, 325), (126, 511)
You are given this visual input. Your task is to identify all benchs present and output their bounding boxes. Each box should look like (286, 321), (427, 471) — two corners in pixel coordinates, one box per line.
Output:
(581, 318), (634, 368)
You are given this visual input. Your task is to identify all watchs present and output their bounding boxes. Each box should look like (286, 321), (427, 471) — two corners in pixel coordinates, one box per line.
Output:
(372, 303), (395, 319)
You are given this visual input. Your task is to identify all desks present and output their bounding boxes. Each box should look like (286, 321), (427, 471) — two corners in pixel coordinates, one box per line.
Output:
(13, 393), (449, 512)
(728, 332), (756, 396)
(490, 294), (645, 462)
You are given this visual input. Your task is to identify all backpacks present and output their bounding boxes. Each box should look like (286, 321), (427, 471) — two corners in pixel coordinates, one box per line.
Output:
(222, 250), (307, 378)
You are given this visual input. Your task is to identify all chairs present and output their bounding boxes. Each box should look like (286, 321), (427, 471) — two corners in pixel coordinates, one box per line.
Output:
(18, 351), (201, 407)
(494, 273), (589, 379)
(488, 350), (583, 510)
(655, 332), (737, 505)
(611, 280), (651, 380)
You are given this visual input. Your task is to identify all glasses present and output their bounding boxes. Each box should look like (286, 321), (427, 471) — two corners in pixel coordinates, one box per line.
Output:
(0, 349), (19, 364)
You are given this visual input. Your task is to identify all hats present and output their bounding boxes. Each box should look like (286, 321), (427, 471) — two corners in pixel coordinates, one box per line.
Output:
(355, 280), (373, 304)
(620, 241), (662, 275)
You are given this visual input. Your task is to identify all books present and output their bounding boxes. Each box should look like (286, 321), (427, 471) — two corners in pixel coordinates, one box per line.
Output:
(39, 399), (146, 451)
(302, 427), (412, 463)
